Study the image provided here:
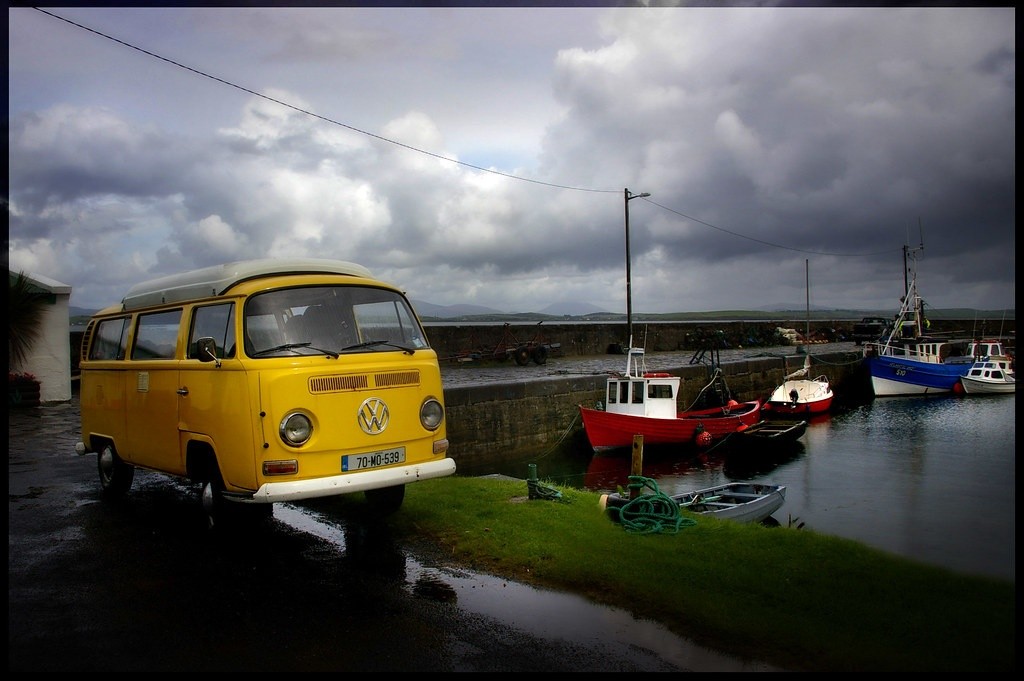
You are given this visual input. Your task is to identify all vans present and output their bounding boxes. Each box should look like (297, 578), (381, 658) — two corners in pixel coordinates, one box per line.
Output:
(77, 258), (458, 522)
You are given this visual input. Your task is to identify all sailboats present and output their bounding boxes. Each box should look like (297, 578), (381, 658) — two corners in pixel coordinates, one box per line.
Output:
(761, 258), (836, 420)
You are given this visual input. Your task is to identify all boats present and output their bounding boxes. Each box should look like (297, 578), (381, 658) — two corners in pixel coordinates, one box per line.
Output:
(577, 326), (761, 456)
(960, 355), (1015, 395)
(666, 481), (787, 525)
(861, 219), (980, 399)
(961, 310), (1008, 360)
(738, 418), (809, 455)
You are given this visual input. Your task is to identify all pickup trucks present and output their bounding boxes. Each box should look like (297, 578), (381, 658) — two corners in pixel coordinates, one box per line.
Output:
(853, 317), (893, 346)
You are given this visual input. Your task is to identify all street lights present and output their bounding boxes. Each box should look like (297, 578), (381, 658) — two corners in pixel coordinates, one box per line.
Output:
(624, 187), (651, 352)
